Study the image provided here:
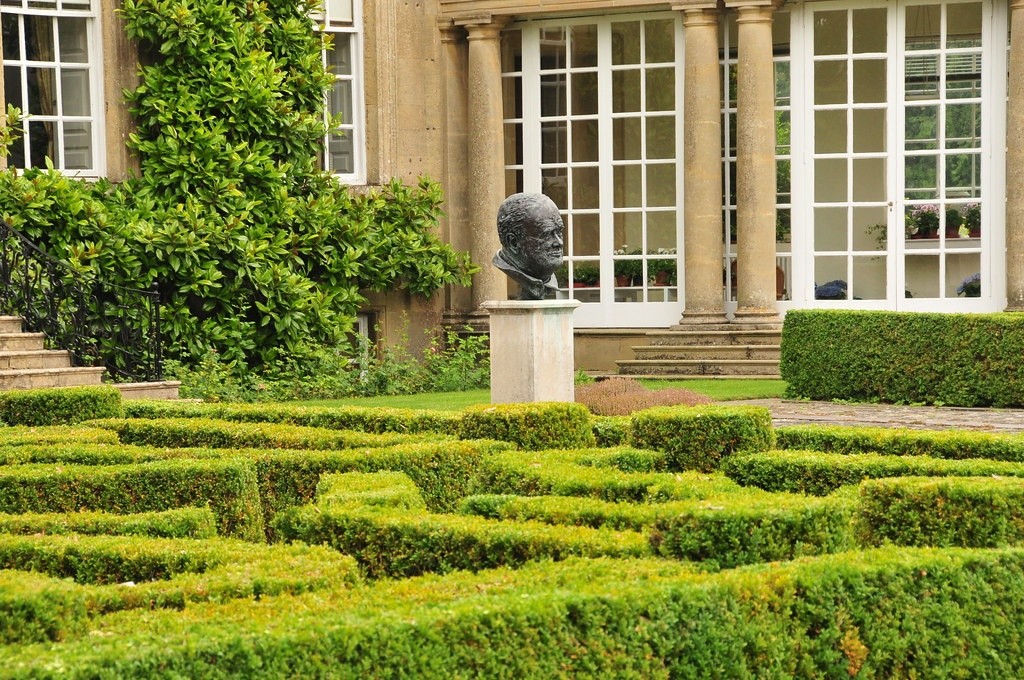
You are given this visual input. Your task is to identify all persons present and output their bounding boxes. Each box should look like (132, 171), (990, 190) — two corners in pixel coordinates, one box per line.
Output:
(492, 192), (565, 298)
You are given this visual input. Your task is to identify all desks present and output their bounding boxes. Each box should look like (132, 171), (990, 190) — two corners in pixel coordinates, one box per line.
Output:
(879, 237), (981, 249)
(556, 286), (678, 302)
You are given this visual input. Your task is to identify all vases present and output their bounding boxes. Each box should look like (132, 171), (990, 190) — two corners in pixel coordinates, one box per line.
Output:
(911, 232), (925, 238)
(616, 276), (632, 287)
(945, 227), (959, 237)
(632, 276), (643, 286)
(654, 270), (673, 284)
(967, 226), (980, 238)
(595, 281), (600, 287)
(925, 228), (940, 239)
(567, 281), (586, 289)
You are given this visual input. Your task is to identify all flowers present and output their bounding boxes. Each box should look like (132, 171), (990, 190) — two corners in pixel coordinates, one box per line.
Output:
(865, 202), (981, 237)
(555, 241), (678, 286)
